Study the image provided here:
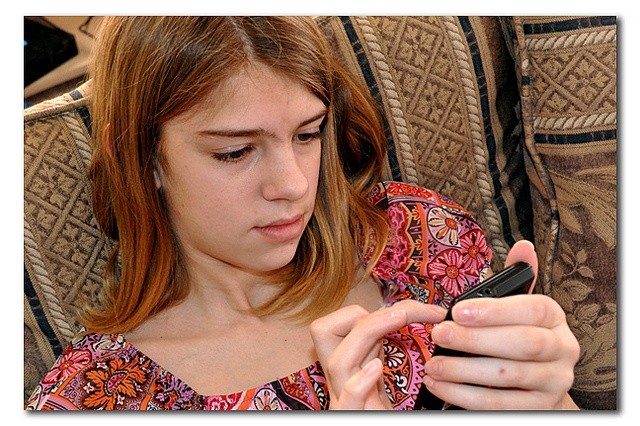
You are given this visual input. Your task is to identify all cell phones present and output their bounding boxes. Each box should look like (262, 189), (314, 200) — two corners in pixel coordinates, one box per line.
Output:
(412, 262), (535, 410)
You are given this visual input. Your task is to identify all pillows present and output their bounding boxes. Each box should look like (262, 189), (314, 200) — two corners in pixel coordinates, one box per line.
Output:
(509, 17), (618, 411)
